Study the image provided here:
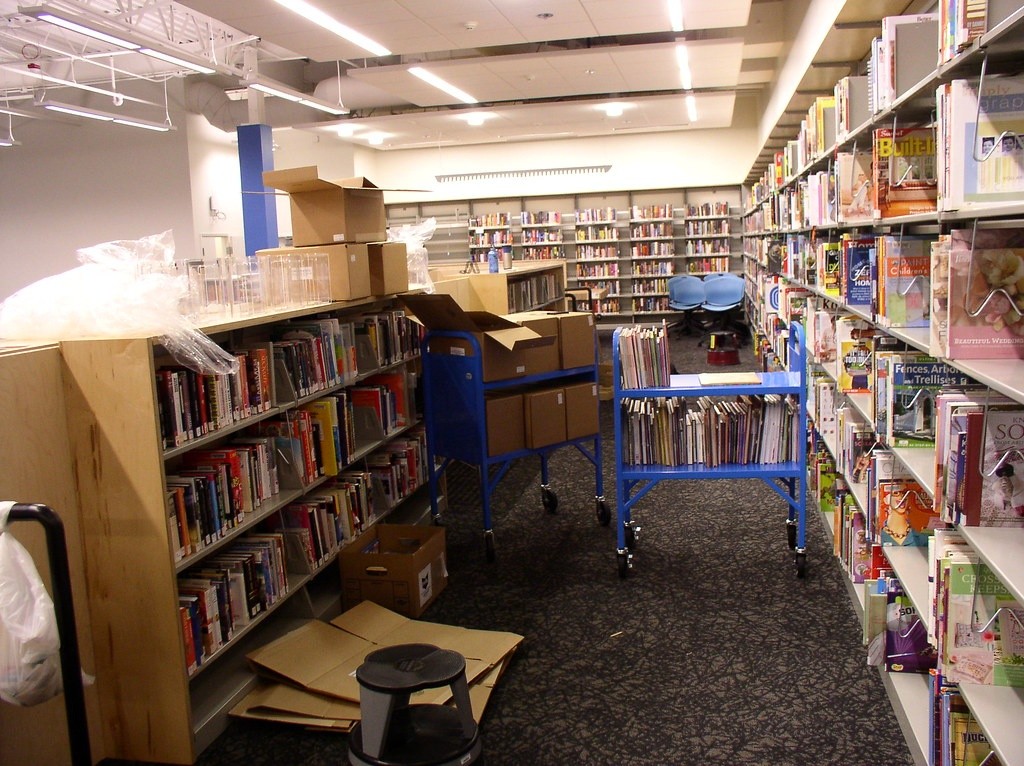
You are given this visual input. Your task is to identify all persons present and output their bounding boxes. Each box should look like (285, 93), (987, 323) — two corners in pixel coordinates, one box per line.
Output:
(1002, 136), (1015, 153)
(982, 137), (994, 155)
(1016, 135), (1024, 150)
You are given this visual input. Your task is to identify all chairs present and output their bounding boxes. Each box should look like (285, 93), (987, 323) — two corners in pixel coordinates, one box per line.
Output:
(667, 273), (753, 350)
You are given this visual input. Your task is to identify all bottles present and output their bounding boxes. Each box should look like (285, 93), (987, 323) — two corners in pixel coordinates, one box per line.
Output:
(488, 247), (499, 272)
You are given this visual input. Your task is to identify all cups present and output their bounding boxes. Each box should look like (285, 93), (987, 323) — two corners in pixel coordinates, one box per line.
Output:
(502, 245), (513, 270)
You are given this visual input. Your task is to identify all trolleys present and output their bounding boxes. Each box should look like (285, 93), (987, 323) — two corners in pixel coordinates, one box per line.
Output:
(612, 322), (806, 577)
(421, 324), (611, 561)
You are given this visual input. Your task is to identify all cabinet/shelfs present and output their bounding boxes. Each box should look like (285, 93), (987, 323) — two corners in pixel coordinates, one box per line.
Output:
(611, 321), (805, 574)
(419, 325), (615, 565)
(743, 0), (1024, 766)
(59, 259), (567, 765)
(467, 200), (743, 314)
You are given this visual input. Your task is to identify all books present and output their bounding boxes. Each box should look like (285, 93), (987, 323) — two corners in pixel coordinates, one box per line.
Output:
(620, 396), (799, 468)
(741, 0), (1024, 766)
(156, 310), (431, 676)
(468, 201), (729, 314)
(618, 318), (760, 390)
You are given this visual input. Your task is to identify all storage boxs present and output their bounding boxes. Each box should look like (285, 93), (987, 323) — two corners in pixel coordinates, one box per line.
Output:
(340, 523), (449, 620)
(396, 294), (601, 459)
(367, 242), (409, 298)
(261, 165), (405, 247)
(254, 239), (372, 301)
